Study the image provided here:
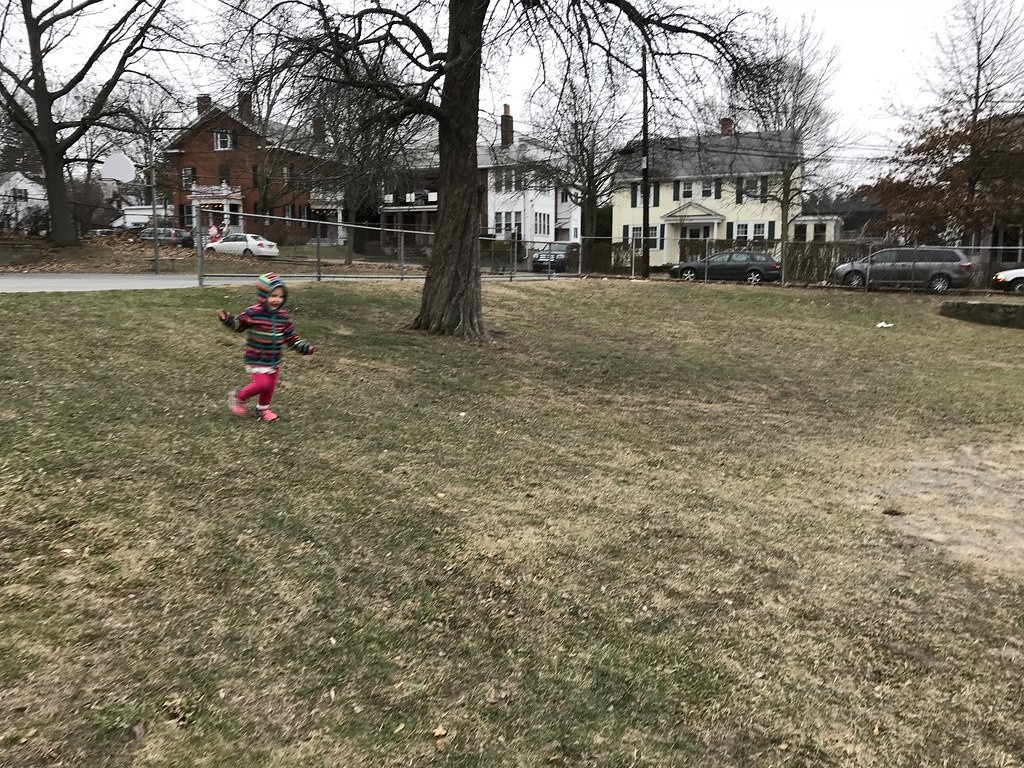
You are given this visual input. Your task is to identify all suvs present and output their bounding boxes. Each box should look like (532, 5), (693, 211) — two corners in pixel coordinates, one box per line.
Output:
(532, 241), (580, 273)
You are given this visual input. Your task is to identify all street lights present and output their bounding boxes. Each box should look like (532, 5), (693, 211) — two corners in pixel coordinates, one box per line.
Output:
(134, 163), (158, 276)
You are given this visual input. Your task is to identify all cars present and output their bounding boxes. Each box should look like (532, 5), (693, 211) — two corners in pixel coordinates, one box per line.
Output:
(992, 269), (1024, 296)
(203, 233), (279, 259)
(668, 252), (782, 286)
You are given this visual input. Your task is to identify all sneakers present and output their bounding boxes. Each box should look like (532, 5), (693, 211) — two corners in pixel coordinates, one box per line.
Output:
(227, 391), (246, 416)
(256, 404), (279, 422)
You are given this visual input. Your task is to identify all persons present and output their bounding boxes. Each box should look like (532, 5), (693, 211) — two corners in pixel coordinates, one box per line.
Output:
(210, 228), (222, 242)
(172, 230), (177, 247)
(216, 272), (315, 422)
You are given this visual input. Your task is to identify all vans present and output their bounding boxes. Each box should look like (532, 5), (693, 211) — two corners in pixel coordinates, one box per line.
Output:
(832, 247), (973, 295)
(92, 230), (114, 237)
(136, 227), (195, 248)
